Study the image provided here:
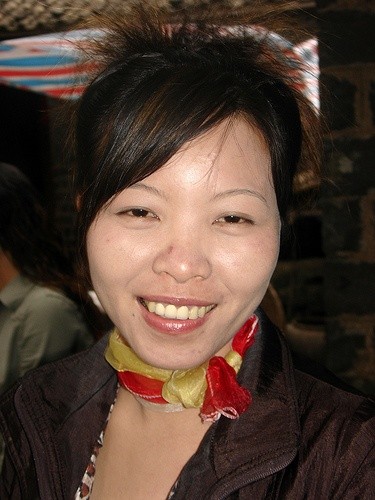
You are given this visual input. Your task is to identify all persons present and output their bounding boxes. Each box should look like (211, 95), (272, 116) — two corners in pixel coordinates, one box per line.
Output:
(1, 2), (375, 500)
(0, 163), (97, 388)
